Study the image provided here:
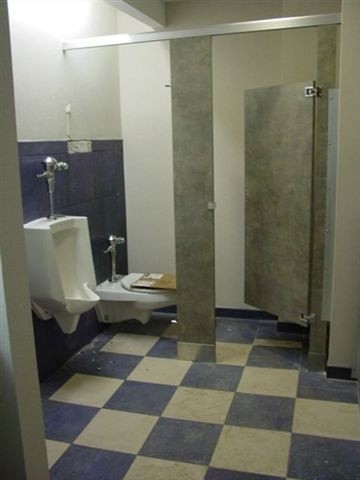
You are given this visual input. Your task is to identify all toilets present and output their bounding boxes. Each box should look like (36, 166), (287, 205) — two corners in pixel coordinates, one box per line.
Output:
(94, 273), (177, 325)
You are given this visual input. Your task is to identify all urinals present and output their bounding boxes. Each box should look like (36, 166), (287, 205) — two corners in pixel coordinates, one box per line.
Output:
(23, 214), (101, 334)
